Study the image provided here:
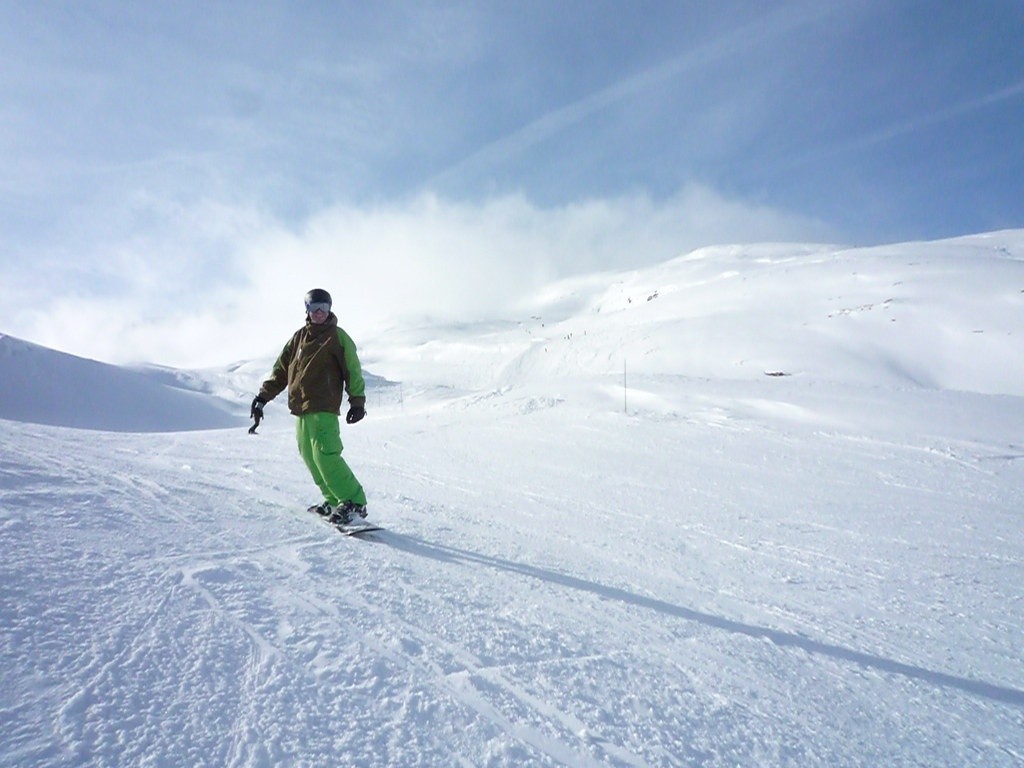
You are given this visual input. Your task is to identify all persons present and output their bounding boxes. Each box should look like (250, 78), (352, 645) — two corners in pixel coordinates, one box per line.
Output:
(251, 289), (367, 522)
(248, 399), (264, 434)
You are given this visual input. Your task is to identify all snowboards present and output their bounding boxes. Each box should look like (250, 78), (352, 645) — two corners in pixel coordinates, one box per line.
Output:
(307, 503), (386, 537)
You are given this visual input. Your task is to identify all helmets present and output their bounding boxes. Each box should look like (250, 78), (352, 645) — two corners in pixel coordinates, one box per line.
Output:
(304, 289), (332, 307)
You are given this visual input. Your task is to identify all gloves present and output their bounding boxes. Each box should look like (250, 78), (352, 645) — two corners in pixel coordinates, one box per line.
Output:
(345, 406), (365, 424)
(249, 397), (267, 419)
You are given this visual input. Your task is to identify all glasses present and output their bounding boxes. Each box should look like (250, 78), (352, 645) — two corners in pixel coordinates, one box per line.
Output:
(307, 303), (331, 313)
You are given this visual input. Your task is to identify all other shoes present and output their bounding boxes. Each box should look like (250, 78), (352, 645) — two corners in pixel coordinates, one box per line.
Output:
(315, 499), (331, 516)
(328, 501), (367, 524)
